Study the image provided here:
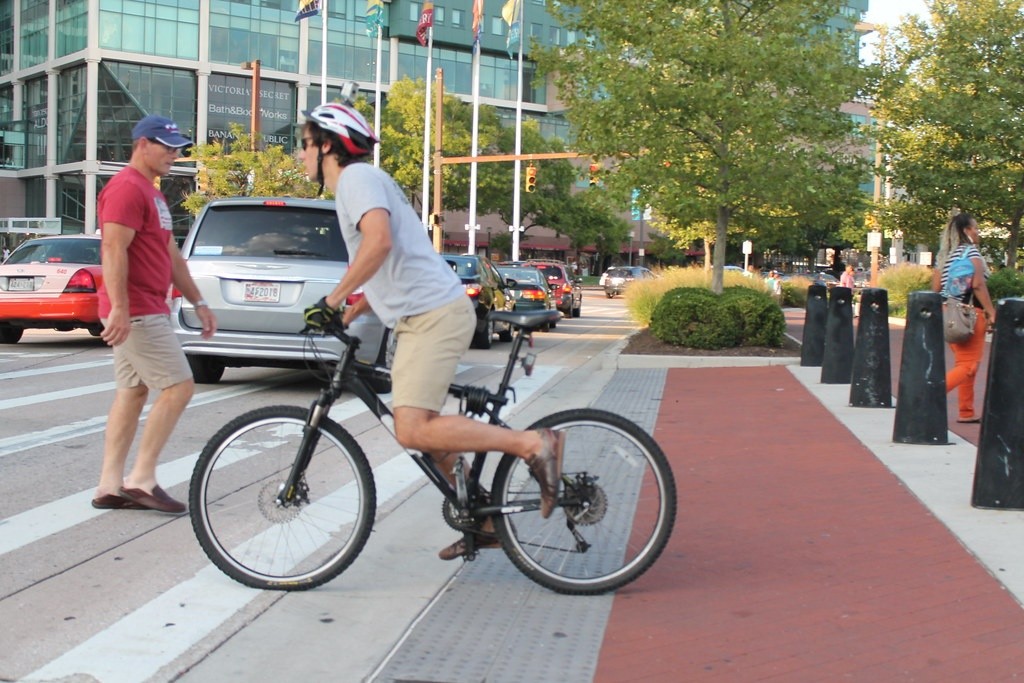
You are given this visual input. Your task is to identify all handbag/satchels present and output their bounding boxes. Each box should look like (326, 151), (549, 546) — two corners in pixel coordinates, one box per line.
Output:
(942, 297), (978, 343)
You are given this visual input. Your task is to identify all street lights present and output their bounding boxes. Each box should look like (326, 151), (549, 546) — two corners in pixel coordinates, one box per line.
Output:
(628, 232), (635, 267)
(239, 60), (261, 151)
(486, 227), (492, 259)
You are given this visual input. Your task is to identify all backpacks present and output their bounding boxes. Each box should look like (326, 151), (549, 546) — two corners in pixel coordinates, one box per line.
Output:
(947, 245), (974, 294)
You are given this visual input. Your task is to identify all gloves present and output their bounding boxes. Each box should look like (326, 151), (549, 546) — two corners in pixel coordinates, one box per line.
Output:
(304, 295), (345, 328)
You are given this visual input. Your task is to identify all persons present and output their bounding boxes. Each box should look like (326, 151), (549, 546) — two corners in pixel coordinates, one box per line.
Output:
(748, 262), (761, 280)
(93, 116), (217, 513)
(773, 275), (781, 305)
(300, 103), (565, 561)
(840, 265), (859, 318)
(2, 247), (10, 261)
(768, 271), (775, 289)
(931, 214), (997, 423)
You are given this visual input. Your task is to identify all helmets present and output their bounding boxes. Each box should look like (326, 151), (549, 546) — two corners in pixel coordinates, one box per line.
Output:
(301, 102), (377, 155)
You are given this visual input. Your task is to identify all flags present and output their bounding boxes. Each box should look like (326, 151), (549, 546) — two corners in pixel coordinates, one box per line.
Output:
(502, 0), (520, 59)
(416, 0), (433, 47)
(472, 0), (484, 55)
(294, 0), (322, 21)
(366, 0), (384, 37)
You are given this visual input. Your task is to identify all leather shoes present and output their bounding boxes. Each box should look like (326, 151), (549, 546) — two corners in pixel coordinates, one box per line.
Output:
(92, 493), (149, 510)
(439, 524), (518, 560)
(119, 484), (185, 514)
(524, 429), (564, 518)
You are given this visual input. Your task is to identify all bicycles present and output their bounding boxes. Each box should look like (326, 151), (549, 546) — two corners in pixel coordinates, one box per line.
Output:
(188, 310), (678, 596)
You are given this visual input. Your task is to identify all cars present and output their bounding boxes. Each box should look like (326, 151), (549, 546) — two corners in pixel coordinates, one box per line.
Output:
(521, 259), (583, 319)
(168, 197), (397, 395)
(496, 267), (559, 332)
(0, 234), (104, 345)
(722, 266), (870, 285)
(442, 255), (518, 350)
(606, 267), (657, 299)
(599, 267), (616, 290)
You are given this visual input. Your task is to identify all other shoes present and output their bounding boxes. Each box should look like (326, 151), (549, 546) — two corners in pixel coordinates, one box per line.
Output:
(957, 414), (981, 423)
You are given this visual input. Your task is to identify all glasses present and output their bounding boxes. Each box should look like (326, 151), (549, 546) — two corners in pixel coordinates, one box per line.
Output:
(148, 138), (182, 154)
(301, 137), (317, 150)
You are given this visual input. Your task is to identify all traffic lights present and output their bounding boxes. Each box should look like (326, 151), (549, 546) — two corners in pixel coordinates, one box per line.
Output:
(589, 164), (599, 188)
(430, 214), (439, 225)
(526, 167), (536, 193)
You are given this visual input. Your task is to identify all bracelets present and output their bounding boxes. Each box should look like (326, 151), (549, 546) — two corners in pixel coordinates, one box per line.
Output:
(194, 299), (208, 309)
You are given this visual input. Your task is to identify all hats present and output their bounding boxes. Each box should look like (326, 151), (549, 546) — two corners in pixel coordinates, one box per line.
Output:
(131, 116), (193, 148)
